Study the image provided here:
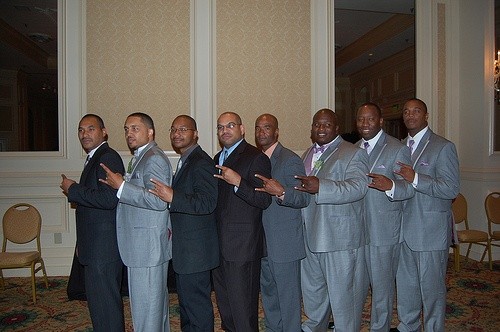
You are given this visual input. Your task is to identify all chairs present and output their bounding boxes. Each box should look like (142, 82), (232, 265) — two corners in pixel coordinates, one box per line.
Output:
(449, 192), (493, 274)
(479, 192), (500, 263)
(0, 202), (50, 306)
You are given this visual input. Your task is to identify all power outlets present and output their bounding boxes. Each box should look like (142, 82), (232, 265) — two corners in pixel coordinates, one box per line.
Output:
(54, 233), (62, 243)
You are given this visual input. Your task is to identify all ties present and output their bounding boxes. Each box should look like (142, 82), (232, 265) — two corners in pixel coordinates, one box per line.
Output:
(408, 140), (415, 153)
(223, 151), (228, 163)
(174, 159), (182, 180)
(84, 155), (90, 168)
(364, 142), (369, 154)
(316, 146), (324, 154)
(132, 149), (138, 165)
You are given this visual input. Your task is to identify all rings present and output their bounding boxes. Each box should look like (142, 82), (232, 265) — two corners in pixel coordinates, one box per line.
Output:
(374, 182), (376, 185)
(301, 183), (305, 188)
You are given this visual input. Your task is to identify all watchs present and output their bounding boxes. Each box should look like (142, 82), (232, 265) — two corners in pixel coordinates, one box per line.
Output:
(281, 191), (286, 196)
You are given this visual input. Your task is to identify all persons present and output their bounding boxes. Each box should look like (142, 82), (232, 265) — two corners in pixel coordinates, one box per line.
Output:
(294, 108), (371, 332)
(388, 99), (460, 332)
(100, 113), (173, 332)
(149, 115), (219, 332)
(212, 112), (272, 332)
(326, 103), (416, 332)
(60, 113), (127, 332)
(253, 114), (311, 332)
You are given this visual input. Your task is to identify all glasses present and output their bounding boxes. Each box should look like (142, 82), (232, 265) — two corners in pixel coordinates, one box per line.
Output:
(168, 128), (192, 133)
(217, 122), (241, 131)
